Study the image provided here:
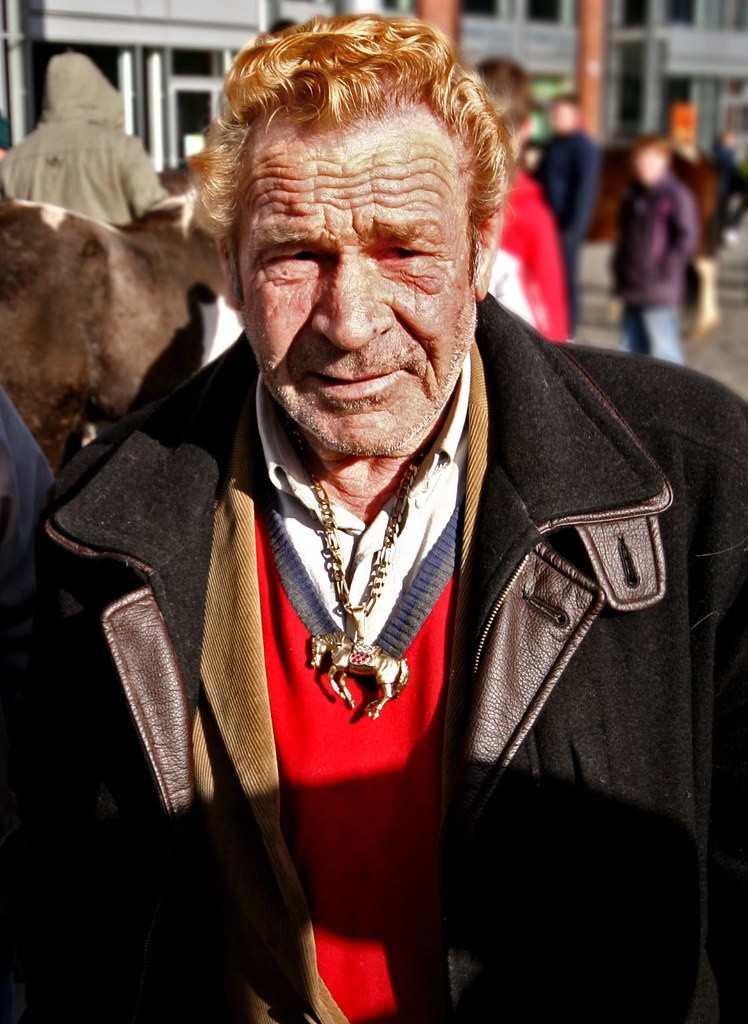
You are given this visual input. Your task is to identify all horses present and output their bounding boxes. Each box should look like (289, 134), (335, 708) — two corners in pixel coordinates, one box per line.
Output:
(309, 631), (409, 720)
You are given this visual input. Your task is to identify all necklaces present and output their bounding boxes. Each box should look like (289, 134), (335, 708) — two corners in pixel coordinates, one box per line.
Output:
(283, 419), (433, 720)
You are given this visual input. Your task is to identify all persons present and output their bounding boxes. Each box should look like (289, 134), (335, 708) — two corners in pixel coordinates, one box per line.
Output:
(0, 11), (746, 1024)
